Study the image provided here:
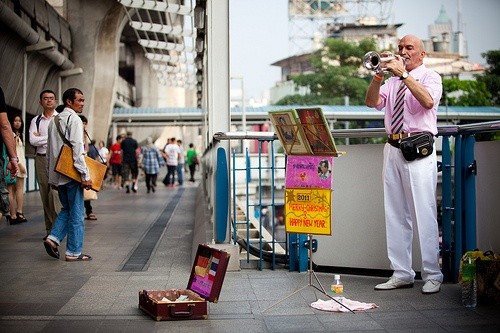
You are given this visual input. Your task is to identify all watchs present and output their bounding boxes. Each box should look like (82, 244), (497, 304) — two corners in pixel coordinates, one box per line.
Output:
(9, 157), (19, 163)
(400, 71), (409, 80)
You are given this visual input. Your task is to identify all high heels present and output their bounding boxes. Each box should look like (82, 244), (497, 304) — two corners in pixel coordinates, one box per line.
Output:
(16, 211), (27, 222)
(6, 215), (18, 225)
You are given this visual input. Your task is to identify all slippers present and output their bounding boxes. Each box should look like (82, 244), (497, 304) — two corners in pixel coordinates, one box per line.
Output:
(66, 253), (92, 261)
(85, 214), (97, 220)
(44, 240), (60, 260)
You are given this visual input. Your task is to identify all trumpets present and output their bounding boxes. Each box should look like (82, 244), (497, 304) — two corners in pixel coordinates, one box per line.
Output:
(363, 49), (408, 76)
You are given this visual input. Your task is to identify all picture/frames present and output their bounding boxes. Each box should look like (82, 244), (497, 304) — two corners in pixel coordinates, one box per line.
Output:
(295, 109), (339, 157)
(267, 110), (311, 156)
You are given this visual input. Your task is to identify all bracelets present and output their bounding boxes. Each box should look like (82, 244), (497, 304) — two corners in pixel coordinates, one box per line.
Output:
(373, 73), (384, 82)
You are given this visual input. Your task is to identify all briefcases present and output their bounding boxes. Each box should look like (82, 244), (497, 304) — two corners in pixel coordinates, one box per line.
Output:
(138, 244), (230, 321)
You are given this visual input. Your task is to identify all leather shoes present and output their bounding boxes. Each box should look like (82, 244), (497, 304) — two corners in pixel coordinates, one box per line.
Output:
(374, 276), (415, 290)
(423, 279), (440, 292)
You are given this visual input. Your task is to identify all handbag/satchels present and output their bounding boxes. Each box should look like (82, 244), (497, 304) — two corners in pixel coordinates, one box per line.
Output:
(388, 133), (434, 162)
(458, 248), (499, 297)
(3, 159), (17, 183)
(191, 149), (199, 165)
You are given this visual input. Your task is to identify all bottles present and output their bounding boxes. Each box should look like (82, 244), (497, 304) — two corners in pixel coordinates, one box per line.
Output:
(330, 274), (343, 309)
(462, 251), (477, 307)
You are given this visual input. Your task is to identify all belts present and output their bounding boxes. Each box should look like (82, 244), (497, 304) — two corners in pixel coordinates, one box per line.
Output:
(388, 133), (415, 139)
(37, 152), (47, 157)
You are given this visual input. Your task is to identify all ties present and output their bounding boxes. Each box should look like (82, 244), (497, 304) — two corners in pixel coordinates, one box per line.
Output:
(391, 80), (407, 134)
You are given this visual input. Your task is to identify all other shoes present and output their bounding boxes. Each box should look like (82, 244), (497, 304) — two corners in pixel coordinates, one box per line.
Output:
(103, 179), (195, 193)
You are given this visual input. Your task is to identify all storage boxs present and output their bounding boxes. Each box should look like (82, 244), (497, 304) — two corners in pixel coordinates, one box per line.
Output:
(138, 244), (230, 320)
(459, 249), (500, 299)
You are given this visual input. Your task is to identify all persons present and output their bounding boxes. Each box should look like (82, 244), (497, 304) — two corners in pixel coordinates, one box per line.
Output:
(28, 90), (63, 241)
(87, 139), (98, 160)
(43, 87), (92, 260)
(161, 138), (185, 186)
(77, 114), (98, 220)
(366, 37), (444, 294)
(107, 133), (123, 190)
(318, 161), (330, 180)
(121, 130), (139, 193)
(140, 136), (168, 194)
(0, 87), (19, 221)
(55, 104), (65, 113)
(3, 113), (27, 222)
(97, 141), (109, 190)
(186, 143), (198, 182)
